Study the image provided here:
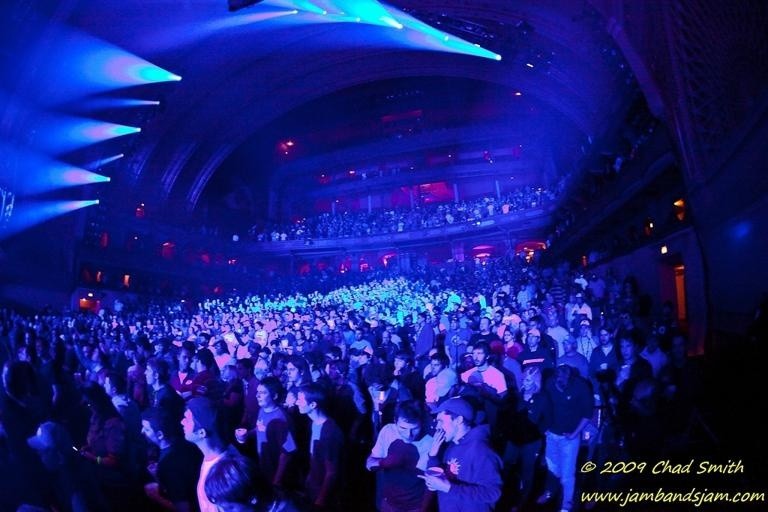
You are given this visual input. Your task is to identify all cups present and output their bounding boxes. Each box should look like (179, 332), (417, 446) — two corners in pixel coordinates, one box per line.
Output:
(147, 463), (157, 475)
(145, 483), (159, 492)
(424, 466), (445, 481)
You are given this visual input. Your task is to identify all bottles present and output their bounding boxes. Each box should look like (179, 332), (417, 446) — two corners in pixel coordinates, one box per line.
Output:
(234, 428), (249, 444)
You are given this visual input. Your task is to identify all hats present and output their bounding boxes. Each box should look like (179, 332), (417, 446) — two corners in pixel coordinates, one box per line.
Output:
(185, 396), (232, 450)
(561, 334), (577, 344)
(429, 397), (473, 420)
(526, 328), (541, 338)
(579, 319), (591, 327)
